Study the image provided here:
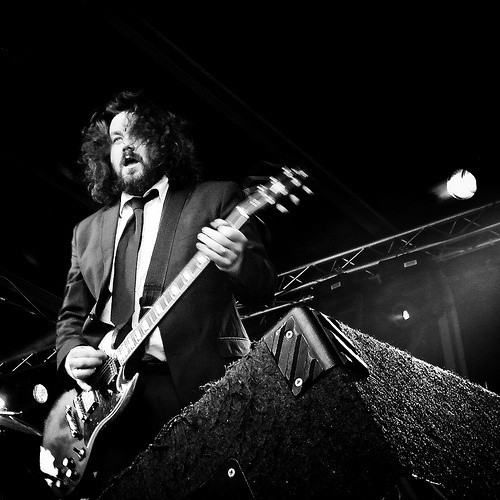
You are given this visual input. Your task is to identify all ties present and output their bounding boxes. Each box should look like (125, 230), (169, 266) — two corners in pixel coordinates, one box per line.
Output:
(110, 190), (157, 330)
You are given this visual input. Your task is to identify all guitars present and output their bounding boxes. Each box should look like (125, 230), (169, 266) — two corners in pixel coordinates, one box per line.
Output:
(38, 165), (314, 496)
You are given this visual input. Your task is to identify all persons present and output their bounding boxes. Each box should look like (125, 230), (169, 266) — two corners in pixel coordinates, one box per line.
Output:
(54, 93), (279, 500)
